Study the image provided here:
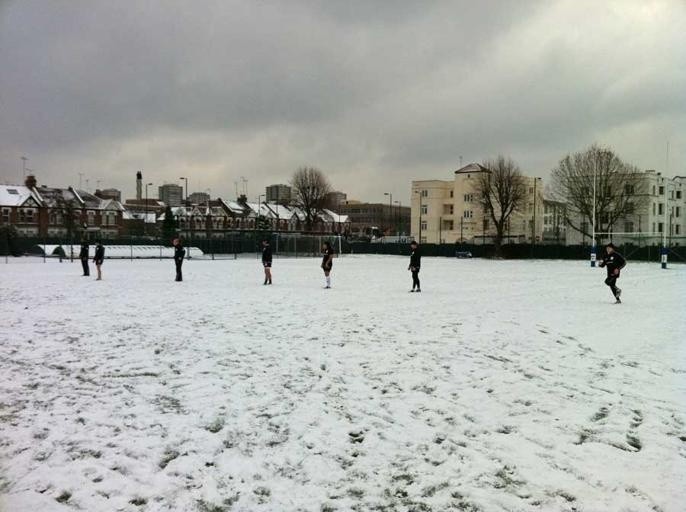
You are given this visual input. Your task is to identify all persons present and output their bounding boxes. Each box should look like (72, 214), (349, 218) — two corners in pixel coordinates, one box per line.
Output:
(79, 236), (90, 275)
(92, 240), (106, 281)
(597, 242), (627, 306)
(262, 239), (272, 285)
(172, 238), (186, 281)
(320, 241), (334, 289)
(407, 241), (423, 293)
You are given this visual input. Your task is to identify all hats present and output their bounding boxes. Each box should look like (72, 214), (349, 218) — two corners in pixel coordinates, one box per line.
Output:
(606, 243), (614, 250)
(410, 241), (417, 245)
(264, 240), (269, 244)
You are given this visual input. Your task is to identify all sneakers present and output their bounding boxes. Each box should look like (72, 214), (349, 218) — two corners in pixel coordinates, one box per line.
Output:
(411, 290), (421, 292)
(613, 288), (621, 304)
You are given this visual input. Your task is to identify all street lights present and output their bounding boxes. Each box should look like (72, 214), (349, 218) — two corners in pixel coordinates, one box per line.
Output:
(395, 201), (401, 222)
(145, 183), (153, 222)
(384, 193), (392, 223)
(533, 177), (541, 242)
(258, 194), (265, 216)
(180, 178), (187, 216)
